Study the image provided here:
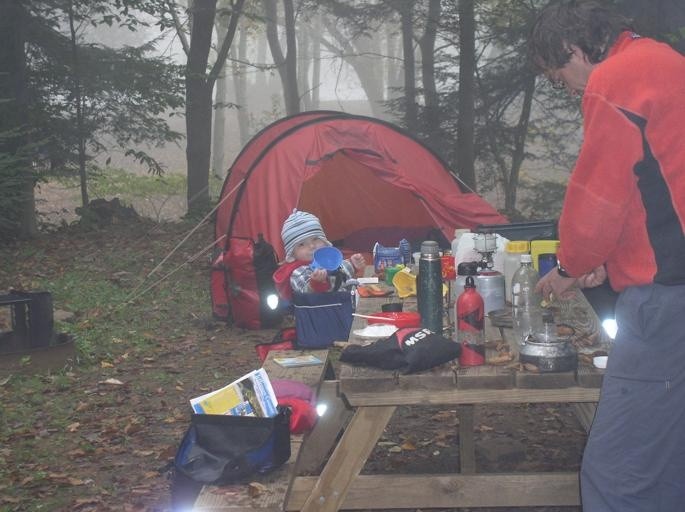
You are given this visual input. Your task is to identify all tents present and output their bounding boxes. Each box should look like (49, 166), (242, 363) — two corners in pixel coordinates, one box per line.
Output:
(209, 111), (516, 334)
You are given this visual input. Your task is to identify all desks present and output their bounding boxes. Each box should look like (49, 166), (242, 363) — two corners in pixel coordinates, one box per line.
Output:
(285, 264), (613, 512)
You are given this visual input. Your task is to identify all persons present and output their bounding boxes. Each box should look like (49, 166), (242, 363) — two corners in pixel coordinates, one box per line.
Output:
(526, 0), (685, 512)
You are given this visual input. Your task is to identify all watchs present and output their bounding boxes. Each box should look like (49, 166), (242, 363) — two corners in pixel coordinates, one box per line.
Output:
(556, 257), (572, 278)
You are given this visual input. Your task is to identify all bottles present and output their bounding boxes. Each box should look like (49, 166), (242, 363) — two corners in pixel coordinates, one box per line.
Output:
(400, 238), (411, 265)
(416, 223), (578, 374)
(378, 259), (385, 278)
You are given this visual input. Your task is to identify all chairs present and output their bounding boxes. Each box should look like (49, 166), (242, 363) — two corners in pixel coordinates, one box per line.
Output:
(0, 289), (76, 356)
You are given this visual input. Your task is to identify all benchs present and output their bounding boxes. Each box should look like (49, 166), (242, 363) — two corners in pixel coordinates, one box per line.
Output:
(193, 347), (335, 512)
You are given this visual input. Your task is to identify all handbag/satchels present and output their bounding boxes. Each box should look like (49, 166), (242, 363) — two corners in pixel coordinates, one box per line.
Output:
(166, 408), (292, 512)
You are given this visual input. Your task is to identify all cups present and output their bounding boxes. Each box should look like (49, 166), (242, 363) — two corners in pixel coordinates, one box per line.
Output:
(382, 303), (403, 313)
(311, 246), (342, 272)
(384, 267), (399, 285)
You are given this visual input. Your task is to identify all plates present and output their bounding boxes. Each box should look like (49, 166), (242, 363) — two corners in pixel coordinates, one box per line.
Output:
(365, 312), (421, 329)
(353, 323), (400, 338)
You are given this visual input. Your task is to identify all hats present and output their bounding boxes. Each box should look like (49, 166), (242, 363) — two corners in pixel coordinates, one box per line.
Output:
(278, 208), (332, 264)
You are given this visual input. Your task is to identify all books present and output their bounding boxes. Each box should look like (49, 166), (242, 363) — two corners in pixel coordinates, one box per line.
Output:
(189, 367), (280, 419)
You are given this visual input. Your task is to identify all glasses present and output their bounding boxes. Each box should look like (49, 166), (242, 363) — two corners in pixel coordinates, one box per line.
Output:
(548, 47), (576, 92)
